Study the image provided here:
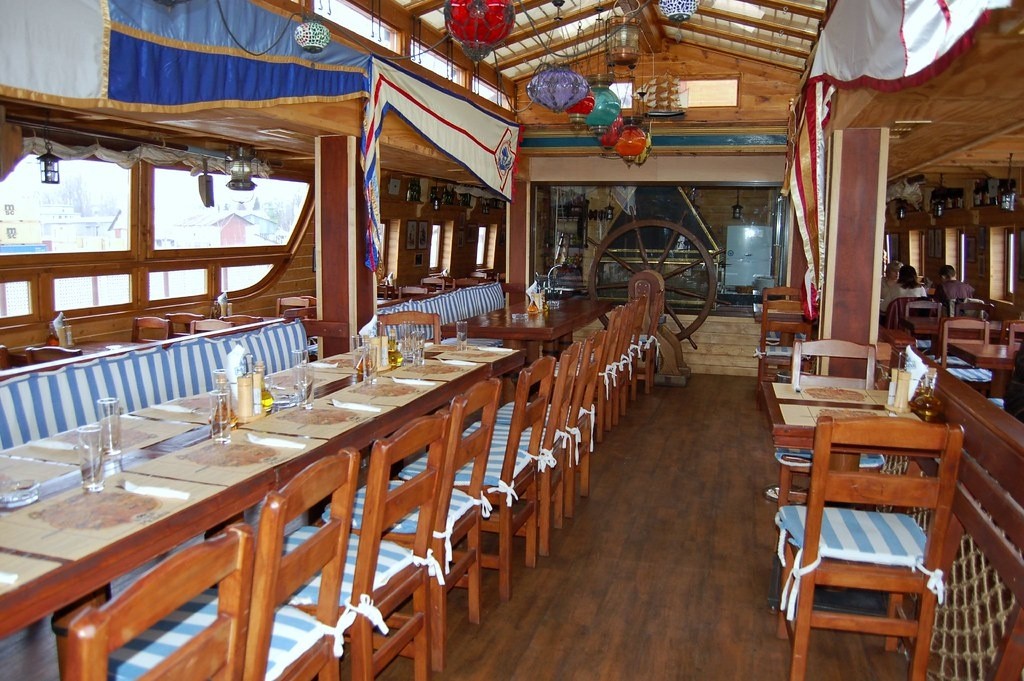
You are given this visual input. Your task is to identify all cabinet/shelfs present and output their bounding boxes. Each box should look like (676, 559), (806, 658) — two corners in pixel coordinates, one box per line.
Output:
(553, 197), (589, 275)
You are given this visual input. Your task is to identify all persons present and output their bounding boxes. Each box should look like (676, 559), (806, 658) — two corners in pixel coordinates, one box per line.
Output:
(880, 266), (927, 312)
(933, 264), (976, 308)
(881, 259), (904, 296)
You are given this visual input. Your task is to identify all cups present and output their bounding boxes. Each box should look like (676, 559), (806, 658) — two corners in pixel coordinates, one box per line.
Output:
(97, 397), (122, 456)
(398, 321), (426, 367)
(290, 349), (308, 396)
(296, 365), (315, 410)
(208, 390), (231, 445)
(362, 344), (377, 384)
(213, 368), (230, 390)
(79, 425), (104, 492)
(457, 321), (468, 353)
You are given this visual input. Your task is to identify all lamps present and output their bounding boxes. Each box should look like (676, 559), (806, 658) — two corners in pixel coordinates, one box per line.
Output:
(407, 181), (491, 215)
(583, 4), (621, 139)
(615, 64), (647, 169)
(731, 191), (743, 219)
(36, 131), (61, 183)
(444, 0), (516, 72)
(607, 15), (641, 67)
(896, 200), (908, 220)
(226, 146), (258, 191)
(526, 0), (590, 115)
(635, 91), (652, 167)
(931, 174), (947, 218)
(293, 15), (331, 54)
(999, 153), (1015, 211)
(604, 204), (615, 221)
(659, 0), (699, 21)
(566, 88), (594, 128)
(600, 115), (623, 150)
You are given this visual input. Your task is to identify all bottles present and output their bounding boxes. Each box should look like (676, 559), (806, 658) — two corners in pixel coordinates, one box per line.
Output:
(354, 328), (403, 384)
(526, 287), (549, 314)
(440, 267), (450, 277)
(551, 188), (590, 266)
(887, 365), (944, 417)
(46, 312), (73, 348)
(210, 292), (232, 319)
(209, 360), (275, 423)
(378, 272), (394, 286)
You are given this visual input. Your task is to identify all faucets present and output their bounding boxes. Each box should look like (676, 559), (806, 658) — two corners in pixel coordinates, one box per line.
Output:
(548, 264), (562, 287)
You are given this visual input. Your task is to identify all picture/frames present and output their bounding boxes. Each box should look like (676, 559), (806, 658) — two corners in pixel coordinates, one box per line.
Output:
(413, 253), (424, 266)
(500, 214), (505, 246)
(979, 227), (985, 249)
(405, 220), (417, 250)
(1018, 228), (1024, 280)
(927, 230), (935, 257)
(966, 237), (977, 263)
(418, 221), (428, 250)
(466, 224), (476, 243)
(459, 212), (466, 229)
(890, 233), (900, 261)
(935, 230), (942, 259)
(458, 232), (464, 247)
(312, 247), (317, 272)
(978, 255), (985, 277)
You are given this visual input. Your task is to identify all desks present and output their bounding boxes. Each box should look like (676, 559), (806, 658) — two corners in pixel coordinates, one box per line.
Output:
(752, 303), (806, 346)
(950, 342), (1019, 397)
(0, 342), (525, 643)
(66, 342), (141, 354)
(902, 317), (1024, 338)
(759, 381), (925, 473)
(438, 299), (612, 358)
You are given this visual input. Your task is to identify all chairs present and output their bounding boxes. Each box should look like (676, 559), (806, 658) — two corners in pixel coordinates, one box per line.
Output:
(776, 338), (885, 505)
(761, 286), (805, 341)
(0, 267), (660, 681)
(757, 300), (810, 381)
(886, 296), (1024, 409)
(773, 416), (966, 680)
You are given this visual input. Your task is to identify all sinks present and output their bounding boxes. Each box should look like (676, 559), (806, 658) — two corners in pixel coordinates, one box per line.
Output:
(544, 287), (574, 295)
